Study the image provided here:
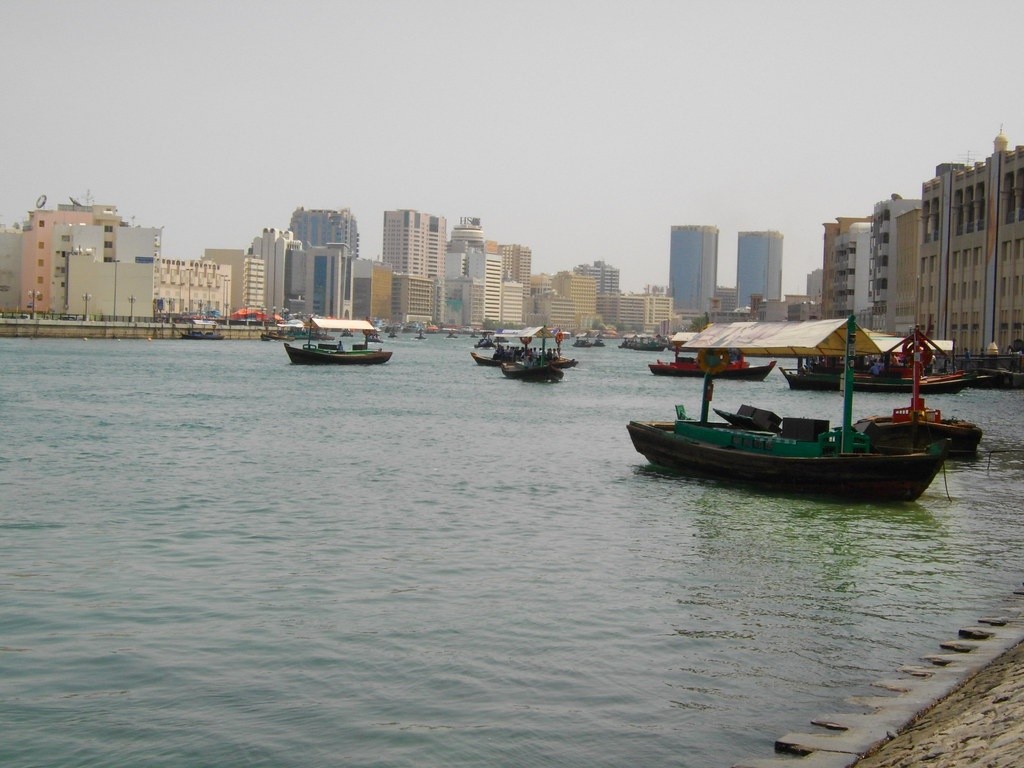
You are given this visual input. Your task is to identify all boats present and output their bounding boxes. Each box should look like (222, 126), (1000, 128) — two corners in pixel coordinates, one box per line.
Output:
(470, 330), (479, 338)
(777, 332), (996, 393)
(498, 323), (579, 377)
(414, 328), (427, 339)
(571, 332), (594, 347)
(472, 331), (496, 349)
(846, 322), (986, 455)
(624, 311), (953, 502)
(617, 333), (675, 352)
(388, 330), (397, 337)
(470, 331), (521, 368)
(446, 330), (458, 338)
(364, 331), (383, 343)
(283, 317), (393, 365)
(591, 333), (607, 347)
(493, 336), (510, 343)
(178, 331), (225, 340)
(649, 330), (779, 381)
(340, 328), (354, 338)
(259, 327), (296, 342)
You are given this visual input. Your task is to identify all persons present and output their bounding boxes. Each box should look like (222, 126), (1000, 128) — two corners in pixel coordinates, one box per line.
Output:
(343, 329), (351, 335)
(496, 345), (557, 368)
(1008, 345), (1017, 372)
(867, 356), (903, 377)
(369, 334), (380, 340)
(931, 354), (937, 373)
(964, 347), (976, 369)
(338, 341), (343, 351)
(479, 339), (491, 346)
(804, 358), (825, 373)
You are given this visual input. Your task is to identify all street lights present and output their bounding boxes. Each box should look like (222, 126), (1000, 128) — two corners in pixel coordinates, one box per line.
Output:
(28, 289), (40, 319)
(82, 293), (93, 318)
(128, 295), (137, 322)
